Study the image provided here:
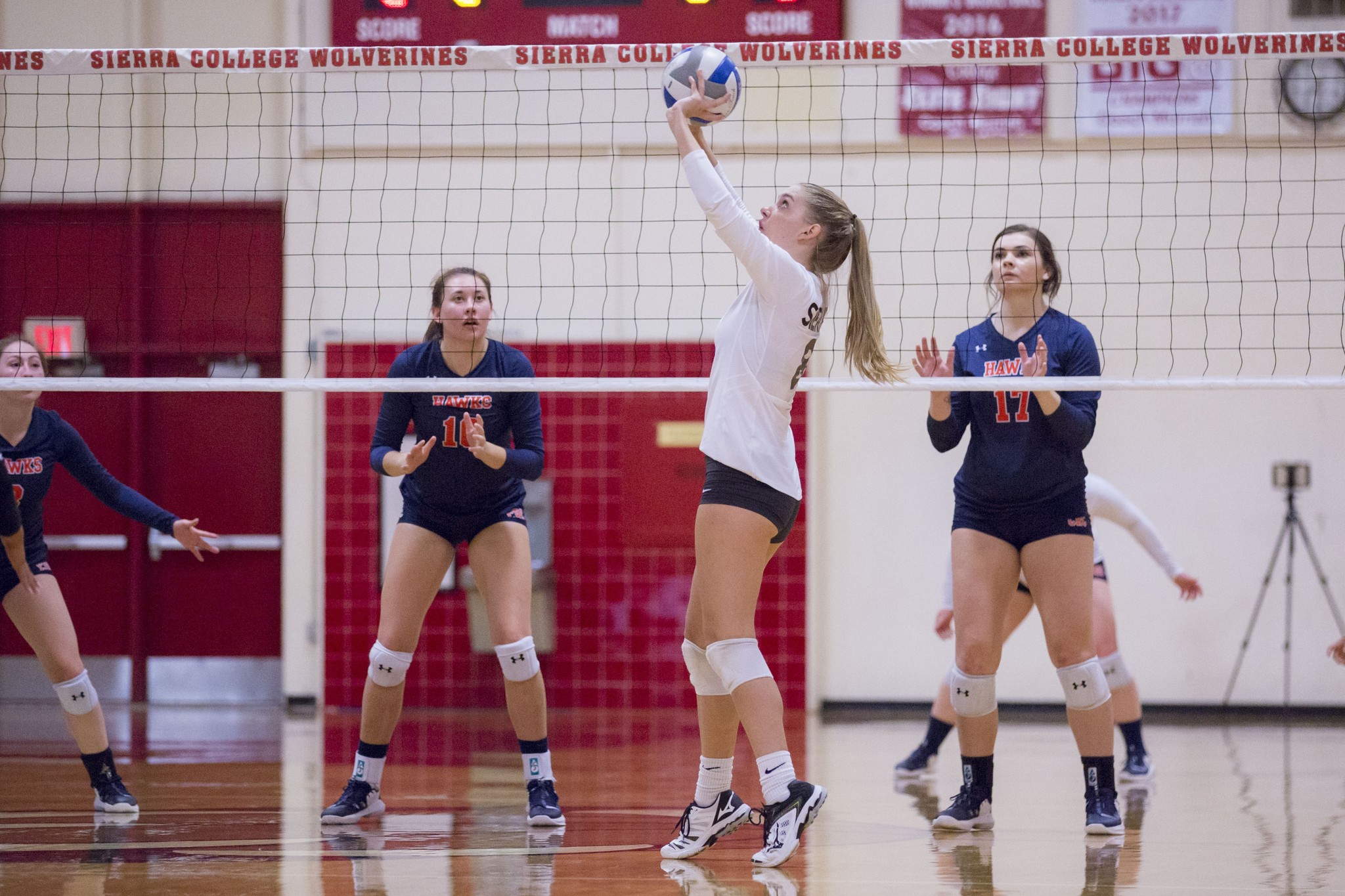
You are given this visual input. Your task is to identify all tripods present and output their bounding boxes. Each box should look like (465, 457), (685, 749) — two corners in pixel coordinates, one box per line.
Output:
(1216, 499), (1345, 720)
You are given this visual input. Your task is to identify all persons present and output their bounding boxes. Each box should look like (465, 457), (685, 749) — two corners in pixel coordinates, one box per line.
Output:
(913, 223), (1124, 832)
(0, 455), (41, 596)
(316, 265), (569, 827)
(0, 333), (221, 812)
(891, 469), (1205, 777)
(660, 66), (910, 868)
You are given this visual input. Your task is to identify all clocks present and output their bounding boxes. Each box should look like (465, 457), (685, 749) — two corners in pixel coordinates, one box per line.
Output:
(1283, 62), (1344, 120)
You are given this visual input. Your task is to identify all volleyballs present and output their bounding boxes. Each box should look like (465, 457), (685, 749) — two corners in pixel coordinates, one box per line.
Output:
(661, 45), (742, 127)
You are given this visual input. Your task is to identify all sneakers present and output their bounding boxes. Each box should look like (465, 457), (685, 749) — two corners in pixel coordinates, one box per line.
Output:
(933, 829), (994, 870)
(896, 743), (940, 776)
(660, 858), (740, 896)
(526, 825), (566, 869)
(1083, 783), (1124, 834)
(660, 789), (754, 860)
(751, 866), (804, 896)
(748, 779), (828, 868)
(895, 773), (940, 806)
(525, 778), (566, 826)
(93, 811), (140, 845)
(320, 774), (386, 825)
(321, 824), (385, 860)
(1124, 785), (1148, 829)
(931, 785), (996, 831)
(1084, 833), (1125, 884)
(91, 773), (140, 812)
(1121, 743), (1149, 780)
